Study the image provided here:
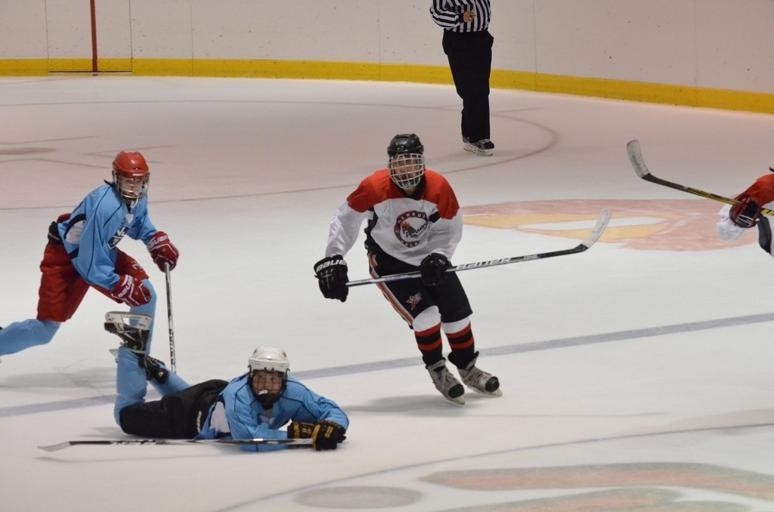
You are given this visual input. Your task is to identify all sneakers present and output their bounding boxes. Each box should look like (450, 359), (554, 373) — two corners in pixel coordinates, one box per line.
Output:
(463, 136), (494, 149)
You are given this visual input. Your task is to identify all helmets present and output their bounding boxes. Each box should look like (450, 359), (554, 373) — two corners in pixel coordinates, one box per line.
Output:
(248, 344), (290, 408)
(112, 150), (150, 200)
(386, 133), (427, 194)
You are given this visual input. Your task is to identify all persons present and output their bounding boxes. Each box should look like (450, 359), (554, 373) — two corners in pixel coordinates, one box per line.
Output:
(310, 130), (500, 404)
(727, 165), (774, 262)
(101, 310), (347, 450)
(0, 147), (180, 366)
(426, 0), (495, 154)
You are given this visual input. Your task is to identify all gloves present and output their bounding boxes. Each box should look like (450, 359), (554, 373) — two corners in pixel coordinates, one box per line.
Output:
(729, 190), (760, 228)
(146, 231), (179, 271)
(312, 420), (346, 450)
(314, 255), (349, 302)
(110, 274), (152, 307)
(421, 253), (452, 285)
(287, 420), (346, 443)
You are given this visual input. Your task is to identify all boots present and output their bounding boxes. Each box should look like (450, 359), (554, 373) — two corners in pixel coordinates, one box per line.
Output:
(422, 355), (465, 398)
(104, 323), (170, 383)
(448, 352), (500, 392)
